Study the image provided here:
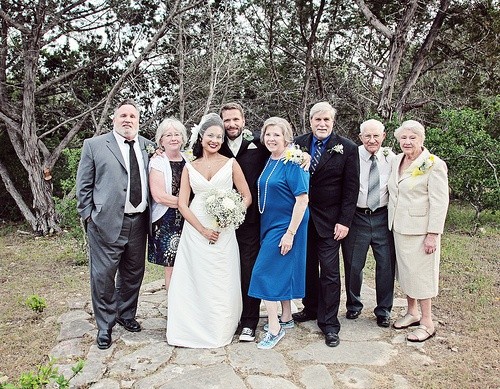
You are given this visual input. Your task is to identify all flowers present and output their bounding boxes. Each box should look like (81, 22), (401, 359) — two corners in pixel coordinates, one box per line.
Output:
(184, 146), (197, 160)
(200, 188), (246, 231)
(384, 146), (393, 156)
(280, 145), (308, 165)
(412, 155), (435, 175)
(242, 128), (254, 141)
(327, 143), (346, 154)
(146, 144), (155, 155)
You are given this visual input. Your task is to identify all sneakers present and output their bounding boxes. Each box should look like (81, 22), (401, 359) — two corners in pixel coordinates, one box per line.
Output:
(263, 318), (295, 330)
(239, 327), (256, 341)
(258, 326), (285, 350)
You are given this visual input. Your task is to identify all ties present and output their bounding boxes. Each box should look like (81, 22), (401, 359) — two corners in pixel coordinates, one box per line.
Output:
(367, 154), (380, 212)
(125, 140), (143, 209)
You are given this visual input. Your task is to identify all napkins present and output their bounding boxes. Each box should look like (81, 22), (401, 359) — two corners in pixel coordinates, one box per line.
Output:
(247, 143), (258, 149)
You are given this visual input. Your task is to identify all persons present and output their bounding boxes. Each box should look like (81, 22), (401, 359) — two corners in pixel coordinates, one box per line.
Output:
(291, 102), (360, 348)
(247, 116), (311, 349)
(165, 113), (253, 349)
(385, 118), (449, 343)
(74, 98), (158, 349)
(148, 118), (196, 296)
(152, 103), (311, 342)
(341, 119), (407, 327)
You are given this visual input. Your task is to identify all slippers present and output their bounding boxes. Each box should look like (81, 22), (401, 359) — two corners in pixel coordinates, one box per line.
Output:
(393, 314), (420, 329)
(407, 324), (435, 342)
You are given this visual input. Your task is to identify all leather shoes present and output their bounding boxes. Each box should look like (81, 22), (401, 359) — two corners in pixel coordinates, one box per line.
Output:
(346, 309), (361, 320)
(324, 331), (340, 347)
(377, 314), (390, 327)
(293, 310), (317, 322)
(97, 328), (113, 350)
(116, 318), (142, 333)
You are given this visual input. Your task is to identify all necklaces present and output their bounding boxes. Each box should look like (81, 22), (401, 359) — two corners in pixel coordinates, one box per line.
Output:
(203, 154), (219, 170)
(257, 143), (288, 215)
